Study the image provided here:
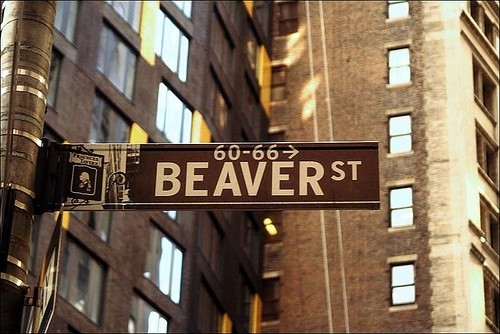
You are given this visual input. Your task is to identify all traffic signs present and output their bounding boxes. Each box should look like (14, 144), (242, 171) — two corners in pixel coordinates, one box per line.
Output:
(33, 136), (381, 212)
